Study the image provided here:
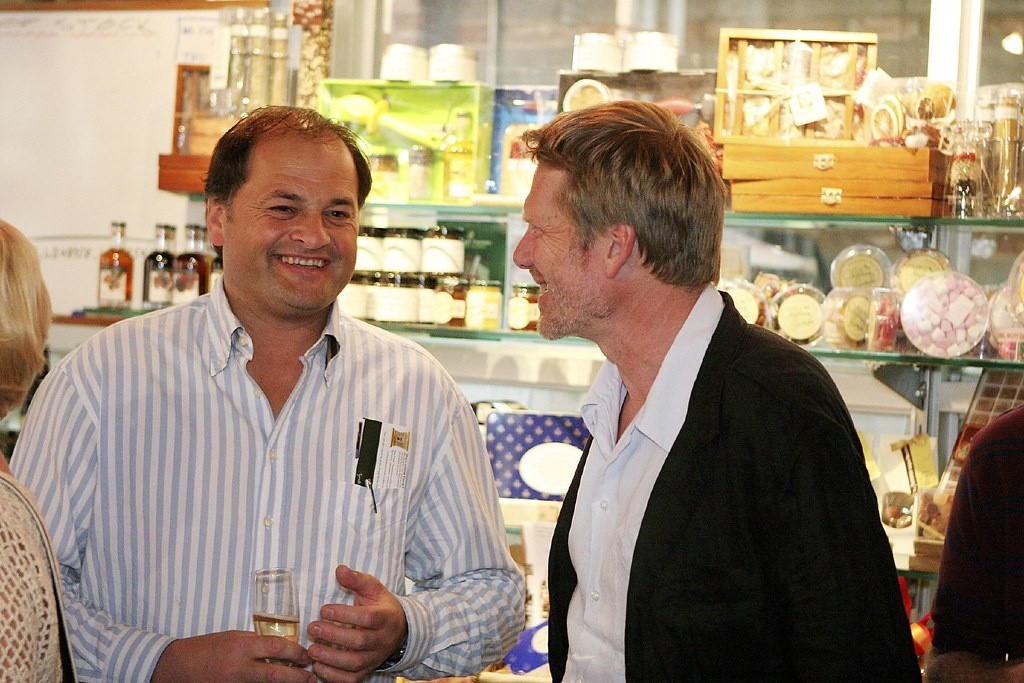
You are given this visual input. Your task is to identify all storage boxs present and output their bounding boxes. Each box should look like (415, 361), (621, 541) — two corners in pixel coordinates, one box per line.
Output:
(170, 0), (953, 218)
(489, 408), (590, 500)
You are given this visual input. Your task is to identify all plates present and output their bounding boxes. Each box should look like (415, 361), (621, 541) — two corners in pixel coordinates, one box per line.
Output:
(900, 273), (989, 357)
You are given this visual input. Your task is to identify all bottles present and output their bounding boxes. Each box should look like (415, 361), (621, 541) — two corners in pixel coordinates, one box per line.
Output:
(143, 223), (224, 306)
(443, 112), (474, 200)
(98, 223), (133, 307)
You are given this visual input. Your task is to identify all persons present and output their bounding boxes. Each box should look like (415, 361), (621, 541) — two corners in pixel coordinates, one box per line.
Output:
(927, 405), (1024, 683)
(9, 106), (526, 683)
(0, 218), (77, 683)
(513, 101), (921, 683)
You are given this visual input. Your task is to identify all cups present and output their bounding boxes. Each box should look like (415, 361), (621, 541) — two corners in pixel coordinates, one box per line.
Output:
(252, 568), (300, 667)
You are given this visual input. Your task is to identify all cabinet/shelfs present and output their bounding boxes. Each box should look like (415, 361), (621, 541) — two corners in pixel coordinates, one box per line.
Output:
(188, 191), (1024, 614)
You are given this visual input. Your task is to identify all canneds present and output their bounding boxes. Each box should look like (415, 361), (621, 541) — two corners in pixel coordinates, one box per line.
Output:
(335, 226), (541, 332)
